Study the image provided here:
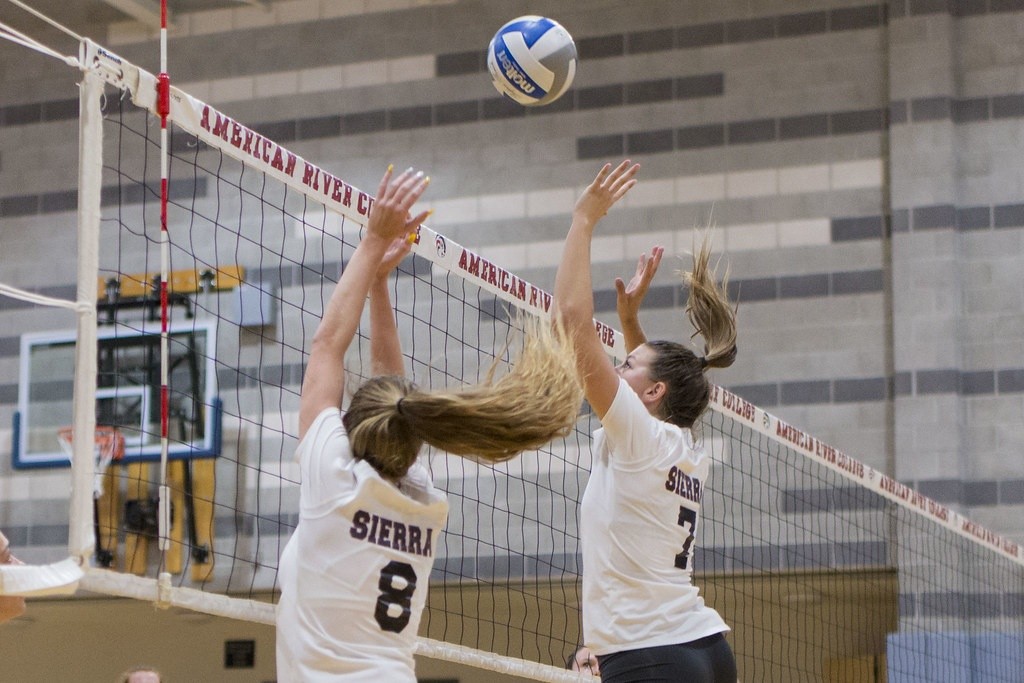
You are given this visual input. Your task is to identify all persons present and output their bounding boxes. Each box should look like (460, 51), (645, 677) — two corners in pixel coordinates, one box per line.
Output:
(0, 529), (29, 625)
(274, 163), (586, 683)
(549, 159), (738, 683)
(567, 645), (601, 677)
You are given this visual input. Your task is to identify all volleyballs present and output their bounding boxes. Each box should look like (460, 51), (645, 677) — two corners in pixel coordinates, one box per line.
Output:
(484, 13), (580, 110)
